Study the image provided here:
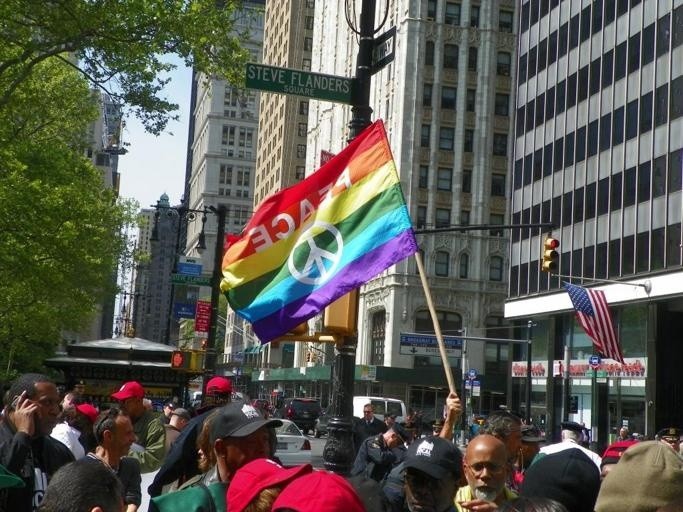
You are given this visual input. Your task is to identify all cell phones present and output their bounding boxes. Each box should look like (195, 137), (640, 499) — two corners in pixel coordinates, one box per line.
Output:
(10, 396), (25, 410)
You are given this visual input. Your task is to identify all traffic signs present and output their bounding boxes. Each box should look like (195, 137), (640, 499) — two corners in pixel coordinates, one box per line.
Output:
(371, 25), (396, 79)
(398, 333), (462, 359)
(171, 272), (211, 286)
(244, 63), (352, 105)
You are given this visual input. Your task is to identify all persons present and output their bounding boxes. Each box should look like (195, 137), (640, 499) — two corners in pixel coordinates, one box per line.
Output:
(0, 375), (683, 512)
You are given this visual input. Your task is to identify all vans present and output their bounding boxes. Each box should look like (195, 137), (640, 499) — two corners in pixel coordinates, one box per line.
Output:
(350, 394), (409, 425)
(192, 391), (242, 410)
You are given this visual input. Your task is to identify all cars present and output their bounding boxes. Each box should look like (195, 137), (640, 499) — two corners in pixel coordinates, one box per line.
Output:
(469, 414), (488, 435)
(269, 417), (312, 468)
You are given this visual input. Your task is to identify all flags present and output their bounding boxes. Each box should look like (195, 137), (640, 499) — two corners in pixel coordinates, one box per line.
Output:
(217, 120), (418, 343)
(564, 279), (625, 366)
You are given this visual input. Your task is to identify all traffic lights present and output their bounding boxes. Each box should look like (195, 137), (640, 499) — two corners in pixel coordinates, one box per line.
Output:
(541, 236), (559, 273)
(306, 353), (310, 362)
(171, 350), (183, 369)
(199, 339), (207, 350)
(311, 353), (316, 362)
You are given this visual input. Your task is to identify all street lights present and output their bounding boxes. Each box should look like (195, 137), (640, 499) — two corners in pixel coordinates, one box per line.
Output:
(148, 200), (229, 404)
(456, 327), (466, 449)
(122, 291), (153, 336)
(113, 315), (127, 337)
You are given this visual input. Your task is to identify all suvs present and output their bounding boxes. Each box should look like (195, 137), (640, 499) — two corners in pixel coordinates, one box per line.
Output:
(250, 399), (269, 418)
(313, 404), (334, 440)
(277, 398), (323, 435)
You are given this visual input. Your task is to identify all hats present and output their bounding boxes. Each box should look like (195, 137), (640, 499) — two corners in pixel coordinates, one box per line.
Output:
(658, 428), (681, 437)
(74, 402), (96, 421)
(209, 402), (366, 512)
(112, 381), (144, 400)
(523, 440), (683, 512)
(399, 434), (462, 479)
(206, 375), (232, 393)
(393, 424), (410, 443)
